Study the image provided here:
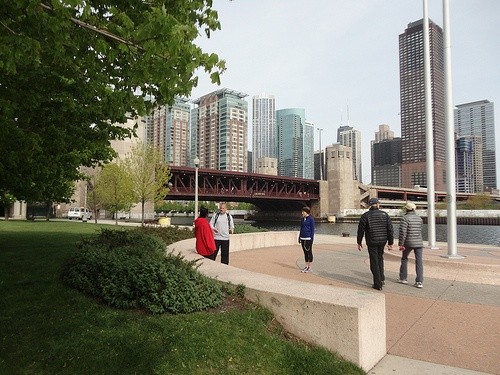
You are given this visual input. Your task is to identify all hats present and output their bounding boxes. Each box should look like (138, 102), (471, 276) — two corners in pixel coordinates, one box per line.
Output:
(303, 207), (310, 214)
(368, 198), (380, 205)
(405, 203), (416, 211)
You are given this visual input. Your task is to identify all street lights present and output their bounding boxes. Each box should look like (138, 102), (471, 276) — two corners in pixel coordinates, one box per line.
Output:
(193, 155), (200, 234)
(392, 164), (400, 188)
(317, 127), (323, 178)
(82, 179), (88, 222)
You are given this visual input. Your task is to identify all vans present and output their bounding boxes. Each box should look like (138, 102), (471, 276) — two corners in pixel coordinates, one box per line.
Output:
(68, 206), (92, 220)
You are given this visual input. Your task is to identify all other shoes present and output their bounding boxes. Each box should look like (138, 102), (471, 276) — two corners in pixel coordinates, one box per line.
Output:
(381, 281), (385, 286)
(304, 267), (311, 273)
(398, 278), (408, 283)
(301, 267), (308, 273)
(414, 282), (423, 287)
(372, 284), (383, 291)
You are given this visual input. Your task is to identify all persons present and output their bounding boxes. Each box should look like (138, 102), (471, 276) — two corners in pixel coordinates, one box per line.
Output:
(357, 197), (394, 290)
(397, 201), (424, 287)
(298, 207), (315, 273)
(210, 203), (234, 265)
(194, 205), (216, 259)
